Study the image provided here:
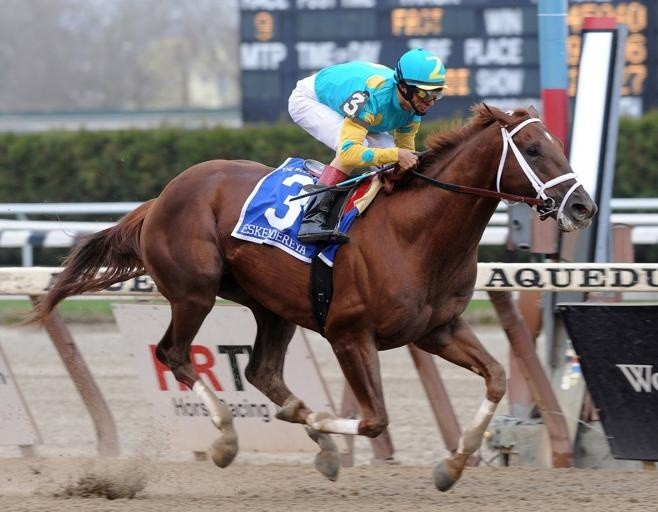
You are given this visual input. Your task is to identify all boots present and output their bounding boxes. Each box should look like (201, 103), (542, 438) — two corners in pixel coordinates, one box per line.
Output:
(297, 164), (350, 244)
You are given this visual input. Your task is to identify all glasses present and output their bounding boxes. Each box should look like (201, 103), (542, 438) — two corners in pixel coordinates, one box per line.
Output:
(415, 90), (444, 100)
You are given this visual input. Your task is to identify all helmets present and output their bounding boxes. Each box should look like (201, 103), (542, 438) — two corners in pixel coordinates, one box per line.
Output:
(393, 48), (449, 90)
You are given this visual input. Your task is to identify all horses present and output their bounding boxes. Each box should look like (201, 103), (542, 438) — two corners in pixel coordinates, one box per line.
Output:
(12, 98), (599, 493)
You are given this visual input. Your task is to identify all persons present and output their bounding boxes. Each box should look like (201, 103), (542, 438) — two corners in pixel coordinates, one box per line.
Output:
(286, 49), (450, 247)
